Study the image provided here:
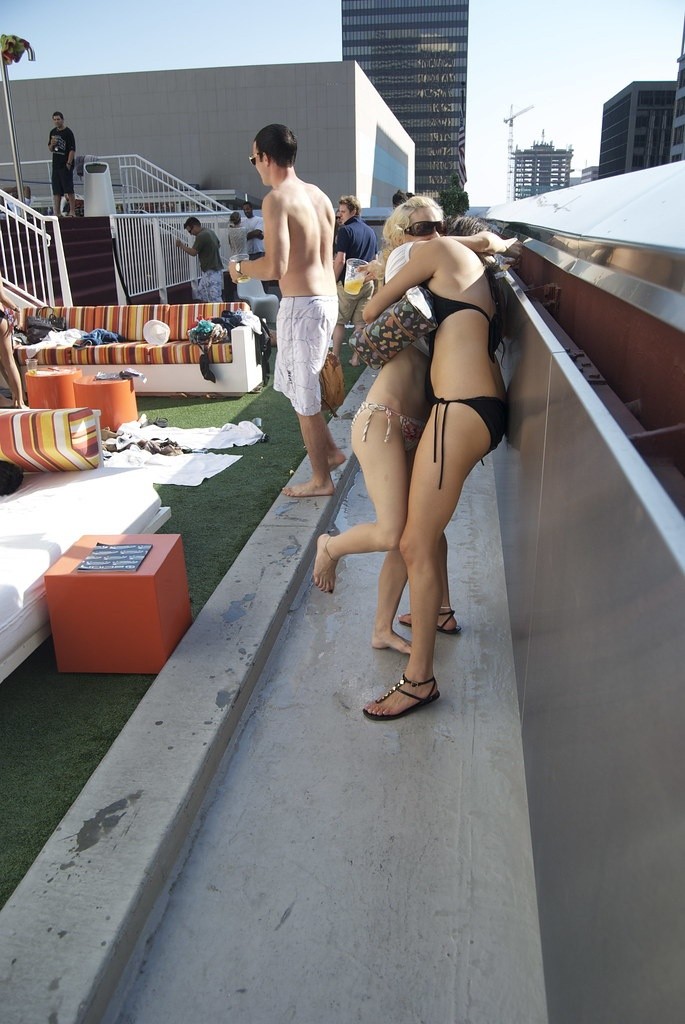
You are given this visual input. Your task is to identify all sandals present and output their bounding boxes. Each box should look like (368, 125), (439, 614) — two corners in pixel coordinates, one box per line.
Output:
(363, 673), (439, 720)
(399, 610), (462, 634)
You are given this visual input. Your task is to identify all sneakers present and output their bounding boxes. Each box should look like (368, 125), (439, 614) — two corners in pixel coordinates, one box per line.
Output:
(146, 438), (192, 456)
(106, 433), (148, 453)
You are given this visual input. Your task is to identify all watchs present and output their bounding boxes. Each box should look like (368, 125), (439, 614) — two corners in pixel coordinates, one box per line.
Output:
(235, 260), (244, 277)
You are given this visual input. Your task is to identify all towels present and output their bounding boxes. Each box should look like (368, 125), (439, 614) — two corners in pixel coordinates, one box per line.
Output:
(115, 413), (264, 450)
(102, 449), (244, 488)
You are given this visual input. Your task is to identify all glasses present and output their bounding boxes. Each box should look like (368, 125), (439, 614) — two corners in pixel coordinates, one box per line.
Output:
(188, 225), (193, 233)
(336, 216), (341, 220)
(404, 221), (446, 236)
(249, 151), (269, 167)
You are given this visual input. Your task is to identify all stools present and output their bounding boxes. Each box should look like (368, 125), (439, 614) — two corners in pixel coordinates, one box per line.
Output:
(42, 534), (193, 675)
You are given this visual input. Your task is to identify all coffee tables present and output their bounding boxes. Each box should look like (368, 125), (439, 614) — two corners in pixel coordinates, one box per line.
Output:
(25, 366), (139, 432)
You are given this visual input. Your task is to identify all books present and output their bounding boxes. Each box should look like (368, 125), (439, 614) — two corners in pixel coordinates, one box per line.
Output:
(93, 367), (147, 384)
(77, 542), (154, 572)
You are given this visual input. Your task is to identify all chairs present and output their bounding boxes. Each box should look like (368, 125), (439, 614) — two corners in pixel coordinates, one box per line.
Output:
(237, 278), (280, 322)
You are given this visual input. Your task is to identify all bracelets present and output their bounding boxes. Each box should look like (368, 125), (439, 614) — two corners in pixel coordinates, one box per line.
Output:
(66, 162), (72, 166)
(13, 305), (19, 312)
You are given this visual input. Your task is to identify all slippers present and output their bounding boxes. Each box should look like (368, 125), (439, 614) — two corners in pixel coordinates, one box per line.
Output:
(140, 417), (153, 428)
(153, 417), (169, 428)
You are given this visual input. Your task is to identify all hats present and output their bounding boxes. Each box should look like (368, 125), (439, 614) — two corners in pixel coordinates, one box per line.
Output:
(143, 320), (170, 345)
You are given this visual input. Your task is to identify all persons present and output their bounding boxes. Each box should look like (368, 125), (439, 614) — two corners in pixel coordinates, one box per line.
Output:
(361, 215), (513, 720)
(0, 271), (31, 409)
(332, 190), (417, 365)
(226, 202), (270, 297)
(228, 124), (346, 497)
(47, 111), (77, 218)
(176, 215), (225, 303)
(311, 193), (523, 659)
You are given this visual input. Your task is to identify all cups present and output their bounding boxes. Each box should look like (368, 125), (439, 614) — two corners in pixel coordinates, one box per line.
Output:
(25, 359), (38, 376)
(344, 258), (369, 295)
(230, 254), (249, 284)
(499, 256), (514, 270)
(51, 136), (58, 145)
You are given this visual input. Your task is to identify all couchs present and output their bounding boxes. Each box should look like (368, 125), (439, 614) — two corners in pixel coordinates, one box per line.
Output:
(13, 301), (263, 398)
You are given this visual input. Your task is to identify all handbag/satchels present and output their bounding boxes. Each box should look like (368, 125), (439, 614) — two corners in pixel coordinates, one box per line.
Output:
(26, 306), (67, 344)
(319, 350), (345, 417)
(348, 285), (439, 370)
(261, 338), (273, 360)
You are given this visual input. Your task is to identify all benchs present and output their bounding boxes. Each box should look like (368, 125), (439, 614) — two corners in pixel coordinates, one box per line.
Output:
(0, 408), (172, 682)
